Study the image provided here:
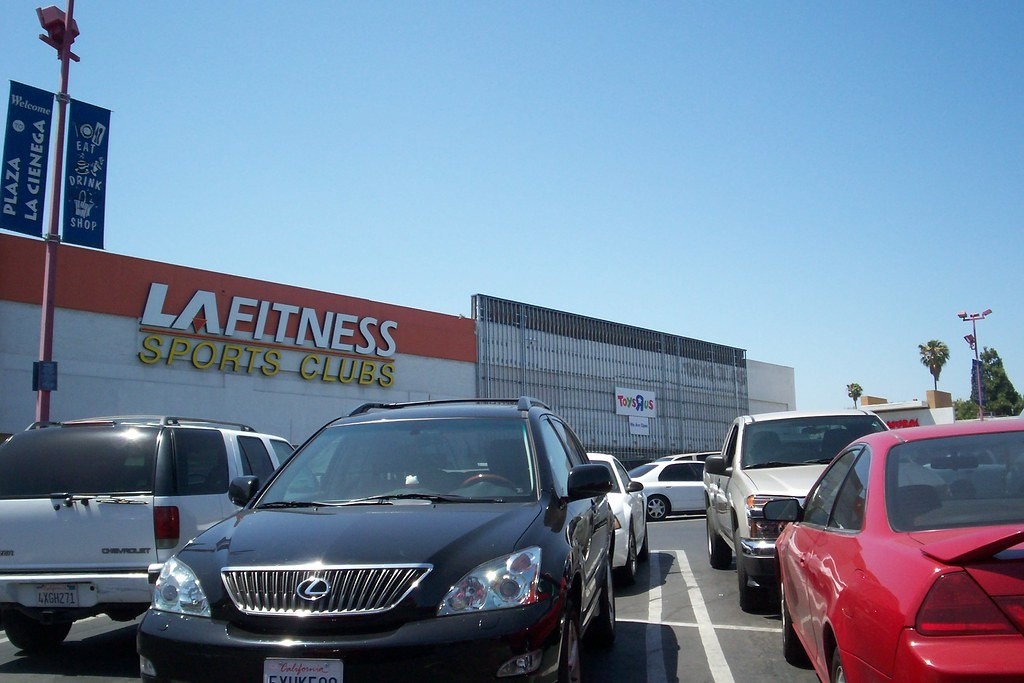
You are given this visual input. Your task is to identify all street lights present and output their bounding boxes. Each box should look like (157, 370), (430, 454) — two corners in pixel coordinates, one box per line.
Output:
(956, 307), (993, 422)
(33, 2), (82, 432)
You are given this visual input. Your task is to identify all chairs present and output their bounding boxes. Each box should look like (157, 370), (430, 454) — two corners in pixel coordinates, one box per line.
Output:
(750, 430), (782, 464)
(820, 427), (854, 460)
(898, 486), (943, 527)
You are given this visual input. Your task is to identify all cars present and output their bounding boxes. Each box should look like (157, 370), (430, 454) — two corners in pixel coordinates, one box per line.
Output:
(627, 460), (706, 521)
(925, 447), (1012, 487)
(581, 452), (649, 578)
(760, 417), (1023, 683)
(654, 450), (722, 481)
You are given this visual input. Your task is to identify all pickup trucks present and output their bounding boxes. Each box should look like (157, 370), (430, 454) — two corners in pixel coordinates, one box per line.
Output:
(699, 408), (949, 612)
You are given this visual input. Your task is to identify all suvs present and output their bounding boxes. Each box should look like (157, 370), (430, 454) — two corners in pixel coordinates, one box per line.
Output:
(141, 396), (620, 683)
(1, 416), (326, 652)
